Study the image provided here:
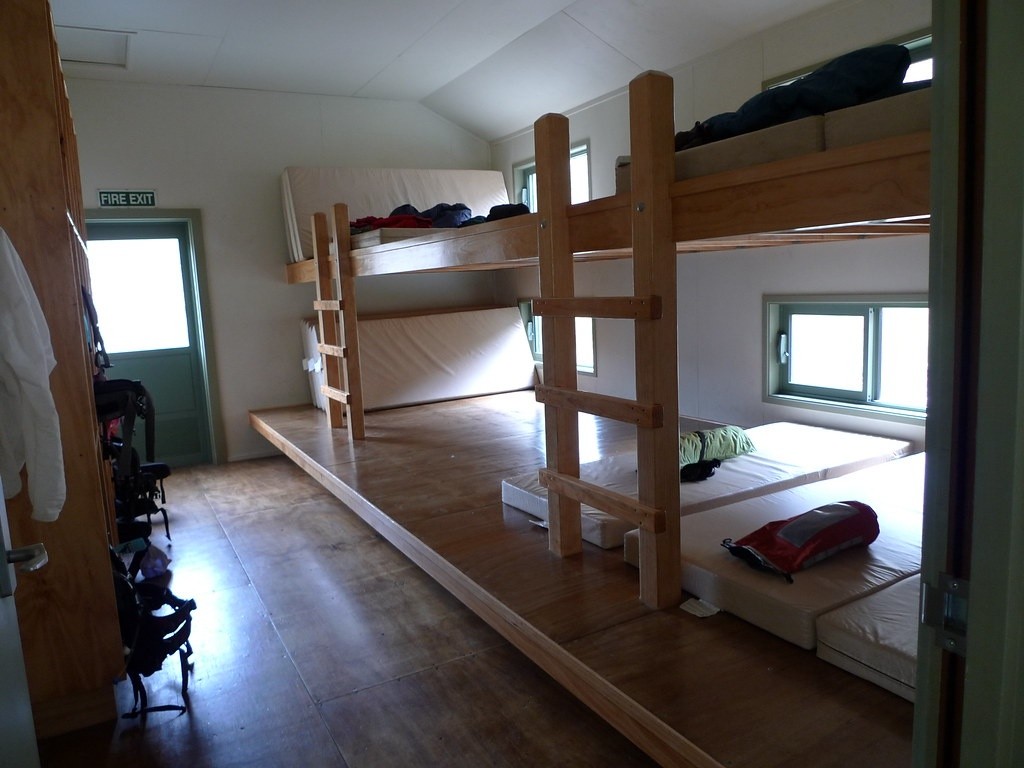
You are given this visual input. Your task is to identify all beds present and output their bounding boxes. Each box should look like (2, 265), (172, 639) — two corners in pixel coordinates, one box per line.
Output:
(815, 573), (921, 703)
(502, 421), (916, 549)
(624, 451), (926, 650)
(616, 115), (825, 193)
(825, 87), (931, 152)
(329, 227), (457, 254)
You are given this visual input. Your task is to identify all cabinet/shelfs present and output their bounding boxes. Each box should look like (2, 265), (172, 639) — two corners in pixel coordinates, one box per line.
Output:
(0, 0), (127, 738)
